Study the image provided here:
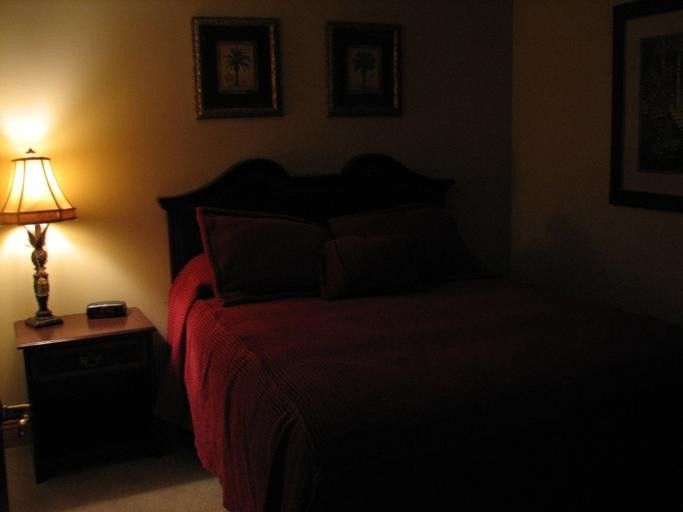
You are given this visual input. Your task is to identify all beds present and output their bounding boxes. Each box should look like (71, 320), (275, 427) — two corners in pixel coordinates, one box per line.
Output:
(143, 148), (682, 509)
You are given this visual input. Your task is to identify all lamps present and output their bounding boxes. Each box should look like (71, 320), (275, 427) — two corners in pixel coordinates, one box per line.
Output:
(0, 141), (79, 334)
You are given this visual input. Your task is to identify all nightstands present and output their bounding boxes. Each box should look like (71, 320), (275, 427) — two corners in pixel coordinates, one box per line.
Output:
(7, 302), (164, 485)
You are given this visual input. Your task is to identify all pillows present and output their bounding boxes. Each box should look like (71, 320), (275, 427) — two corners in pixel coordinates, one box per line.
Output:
(189, 200), (336, 310)
(321, 196), (490, 288)
(303, 225), (436, 304)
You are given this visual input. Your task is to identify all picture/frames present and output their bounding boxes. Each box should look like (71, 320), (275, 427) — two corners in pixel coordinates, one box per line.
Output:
(319, 17), (404, 119)
(189, 12), (285, 121)
(603, 0), (681, 217)
